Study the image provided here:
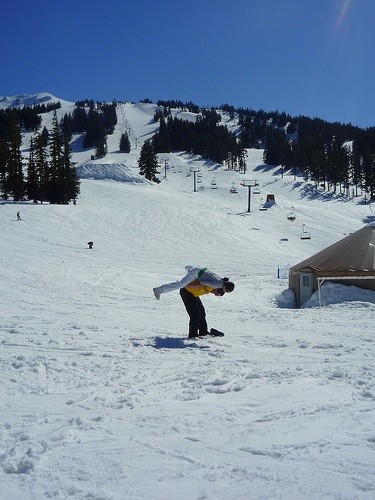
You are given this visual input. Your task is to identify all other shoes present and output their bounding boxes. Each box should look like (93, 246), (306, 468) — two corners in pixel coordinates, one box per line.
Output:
(153, 287), (160, 301)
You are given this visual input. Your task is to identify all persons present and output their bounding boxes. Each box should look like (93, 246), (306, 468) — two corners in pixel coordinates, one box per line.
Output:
(153, 267), (234, 301)
(179, 279), (225, 339)
